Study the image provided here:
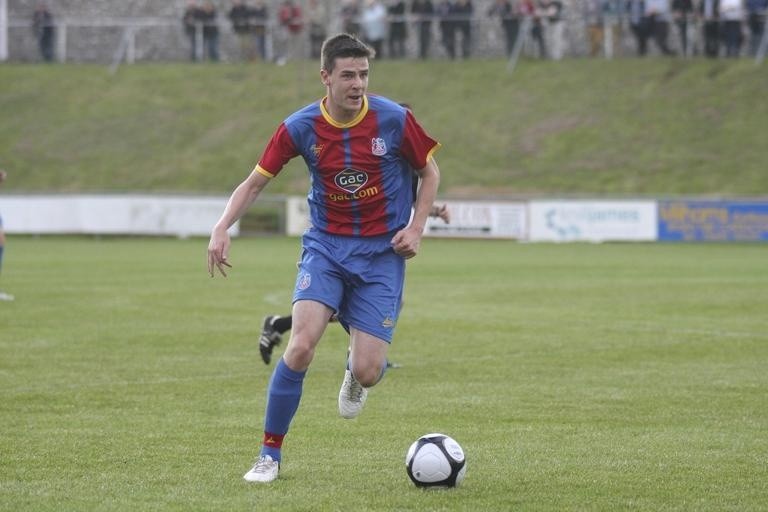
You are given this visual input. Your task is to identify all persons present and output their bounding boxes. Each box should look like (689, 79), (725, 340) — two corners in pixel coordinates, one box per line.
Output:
(33, 4), (55, 62)
(208, 34), (441, 483)
(259, 104), (449, 367)
(182, 1), (768, 60)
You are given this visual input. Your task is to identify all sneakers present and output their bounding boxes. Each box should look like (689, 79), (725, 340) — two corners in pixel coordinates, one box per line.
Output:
(259, 315), (283, 365)
(244, 455), (280, 483)
(339, 346), (368, 419)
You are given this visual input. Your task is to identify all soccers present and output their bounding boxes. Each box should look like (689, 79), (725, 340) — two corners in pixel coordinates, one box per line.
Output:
(405, 433), (465, 489)
